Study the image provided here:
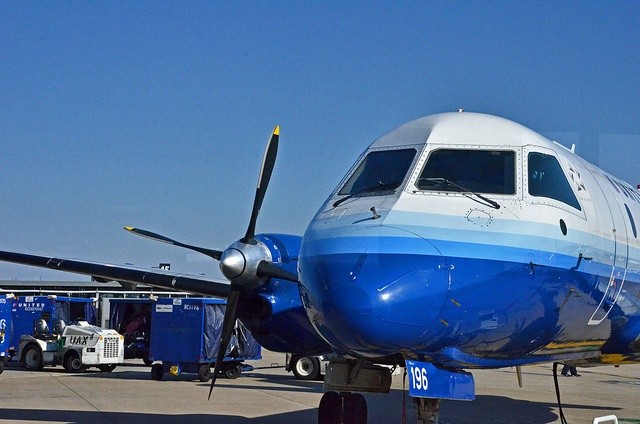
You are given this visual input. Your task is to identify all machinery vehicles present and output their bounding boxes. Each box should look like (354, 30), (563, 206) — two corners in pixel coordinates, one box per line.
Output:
(286, 352), (401, 380)
(18, 318), (125, 372)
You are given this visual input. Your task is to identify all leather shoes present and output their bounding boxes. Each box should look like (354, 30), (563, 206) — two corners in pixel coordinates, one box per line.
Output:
(571, 372), (581, 376)
(561, 373), (572, 377)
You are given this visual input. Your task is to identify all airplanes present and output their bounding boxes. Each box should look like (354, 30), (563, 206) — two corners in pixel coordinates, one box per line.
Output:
(0, 107), (640, 423)
